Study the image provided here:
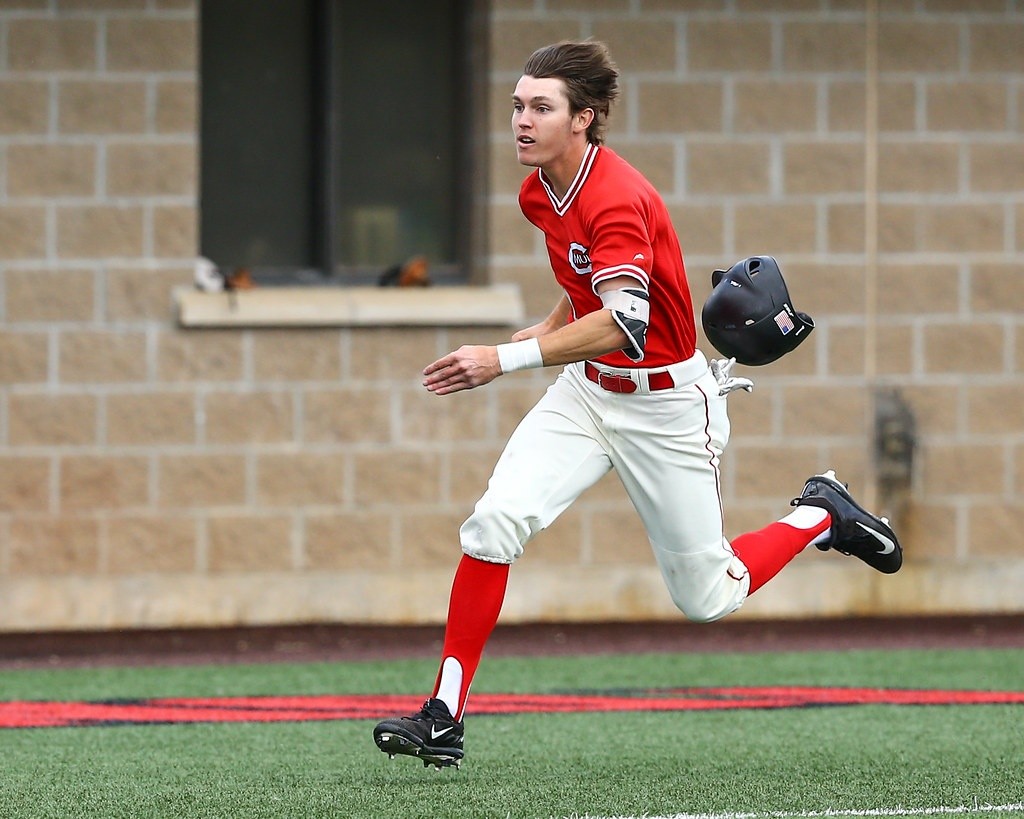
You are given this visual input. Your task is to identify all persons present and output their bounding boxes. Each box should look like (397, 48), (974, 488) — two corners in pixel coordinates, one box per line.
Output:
(374, 38), (902, 769)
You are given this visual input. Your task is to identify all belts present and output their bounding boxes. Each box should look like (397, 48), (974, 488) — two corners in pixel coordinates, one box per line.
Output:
(584, 361), (675, 393)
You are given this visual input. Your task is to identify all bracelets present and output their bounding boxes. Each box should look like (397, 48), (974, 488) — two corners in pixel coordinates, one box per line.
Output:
(497, 338), (543, 373)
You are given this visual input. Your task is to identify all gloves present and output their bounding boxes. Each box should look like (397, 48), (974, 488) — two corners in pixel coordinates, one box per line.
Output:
(709, 357), (753, 397)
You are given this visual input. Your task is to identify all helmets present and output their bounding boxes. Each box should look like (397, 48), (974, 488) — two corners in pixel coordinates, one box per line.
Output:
(701, 255), (816, 366)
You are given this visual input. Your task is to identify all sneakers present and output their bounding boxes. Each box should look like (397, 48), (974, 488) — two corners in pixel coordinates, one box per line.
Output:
(371, 698), (465, 771)
(790, 470), (903, 575)
